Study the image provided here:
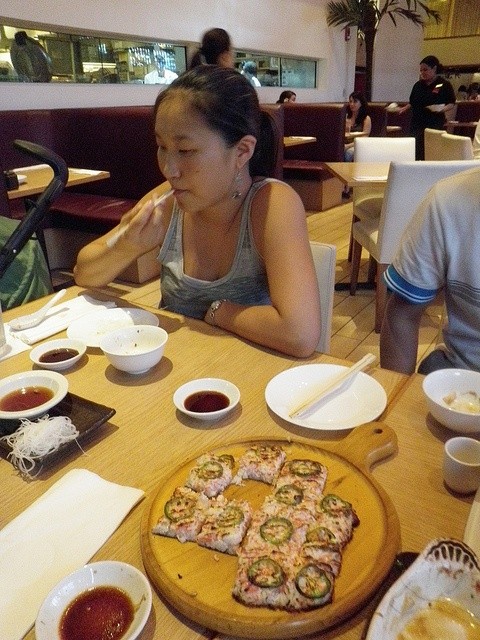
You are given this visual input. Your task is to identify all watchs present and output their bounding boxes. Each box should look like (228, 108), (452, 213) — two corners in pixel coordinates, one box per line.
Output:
(209, 298), (226, 324)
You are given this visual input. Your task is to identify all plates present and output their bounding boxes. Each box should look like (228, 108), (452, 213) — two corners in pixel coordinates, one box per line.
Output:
(267, 365), (387, 430)
(384, 107), (400, 112)
(69, 308), (161, 348)
(367, 537), (480, 639)
(0, 393), (116, 465)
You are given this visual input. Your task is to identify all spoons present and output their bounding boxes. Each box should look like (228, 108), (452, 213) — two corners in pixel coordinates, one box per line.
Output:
(7, 289), (68, 328)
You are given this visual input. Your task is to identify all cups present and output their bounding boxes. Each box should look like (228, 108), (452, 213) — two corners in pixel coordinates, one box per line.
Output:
(0, 302), (8, 356)
(345, 120), (350, 135)
(445, 436), (479, 497)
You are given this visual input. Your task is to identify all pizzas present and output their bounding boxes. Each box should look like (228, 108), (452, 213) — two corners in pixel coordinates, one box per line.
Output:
(184, 453), (235, 496)
(234, 444), (294, 486)
(232, 442), (374, 609)
(153, 486), (256, 555)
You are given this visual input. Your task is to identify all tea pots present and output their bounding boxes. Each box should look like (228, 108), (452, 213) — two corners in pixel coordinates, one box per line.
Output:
(455, 92), (467, 102)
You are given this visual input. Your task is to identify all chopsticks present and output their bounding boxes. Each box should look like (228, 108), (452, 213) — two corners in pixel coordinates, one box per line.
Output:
(287, 353), (375, 418)
(105, 189), (176, 248)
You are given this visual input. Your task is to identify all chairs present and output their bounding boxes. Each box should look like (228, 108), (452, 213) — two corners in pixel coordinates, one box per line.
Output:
(349, 160), (480, 334)
(442, 134), (473, 160)
(423, 128), (447, 161)
(310, 241), (336, 355)
(348, 137), (416, 264)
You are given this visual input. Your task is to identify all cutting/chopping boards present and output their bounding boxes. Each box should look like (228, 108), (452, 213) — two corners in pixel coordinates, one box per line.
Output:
(142, 423), (396, 639)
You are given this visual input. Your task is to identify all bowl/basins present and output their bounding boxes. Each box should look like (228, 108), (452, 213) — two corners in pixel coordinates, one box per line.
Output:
(427, 104), (445, 112)
(31, 338), (86, 370)
(172, 377), (242, 423)
(36, 560), (153, 640)
(98, 326), (168, 374)
(1, 371), (68, 418)
(424, 367), (480, 435)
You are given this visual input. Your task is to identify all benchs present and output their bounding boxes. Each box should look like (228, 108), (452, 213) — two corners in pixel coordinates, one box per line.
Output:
(368, 103), (387, 137)
(1, 104), (285, 282)
(388, 102), (411, 136)
(282, 102), (347, 211)
(442, 100), (480, 143)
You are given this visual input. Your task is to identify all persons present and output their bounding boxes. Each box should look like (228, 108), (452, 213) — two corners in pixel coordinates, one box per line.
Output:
(342, 93), (372, 199)
(240, 60), (261, 88)
(276, 91), (296, 103)
(73, 63), (322, 359)
(379, 165), (479, 375)
(399, 55), (456, 161)
(189, 28), (234, 70)
(144, 50), (179, 84)
